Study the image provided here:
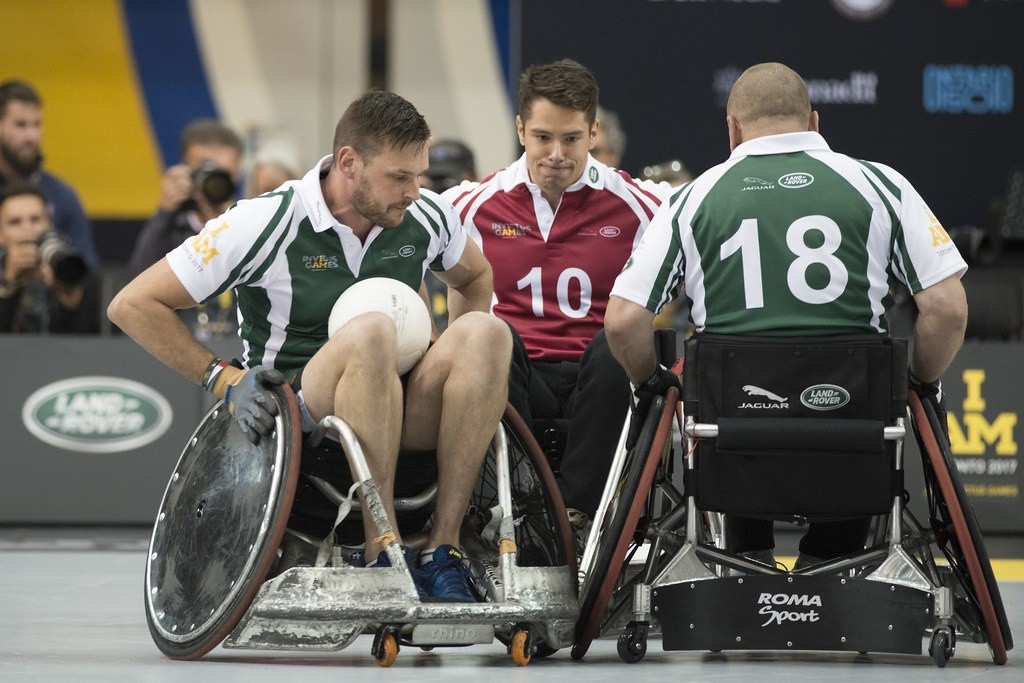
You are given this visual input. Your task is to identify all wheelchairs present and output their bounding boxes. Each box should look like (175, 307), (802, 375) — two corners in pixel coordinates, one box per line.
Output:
(144, 325), (1013, 670)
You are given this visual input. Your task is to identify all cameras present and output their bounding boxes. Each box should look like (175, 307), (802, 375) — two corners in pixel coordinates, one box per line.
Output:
(19, 230), (88, 285)
(179, 160), (234, 211)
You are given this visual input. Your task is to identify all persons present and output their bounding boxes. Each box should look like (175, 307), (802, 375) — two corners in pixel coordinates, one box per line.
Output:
(590, 108), (623, 166)
(106, 88), (514, 603)
(127, 121), (306, 278)
(0, 80), (107, 334)
(429, 133), (473, 190)
(603, 61), (969, 581)
(441, 59), (671, 527)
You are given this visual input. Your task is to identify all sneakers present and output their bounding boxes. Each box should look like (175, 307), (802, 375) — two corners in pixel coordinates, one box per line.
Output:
(416, 544), (485, 603)
(367, 545), (433, 602)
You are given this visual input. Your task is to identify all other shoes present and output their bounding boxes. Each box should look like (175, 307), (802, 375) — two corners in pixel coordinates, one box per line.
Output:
(566, 508), (593, 568)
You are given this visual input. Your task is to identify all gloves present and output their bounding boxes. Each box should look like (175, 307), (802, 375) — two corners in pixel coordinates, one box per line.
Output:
(201, 358), (284, 445)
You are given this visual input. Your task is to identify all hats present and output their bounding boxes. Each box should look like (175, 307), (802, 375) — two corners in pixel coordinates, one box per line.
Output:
(426, 140), (474, 179)
